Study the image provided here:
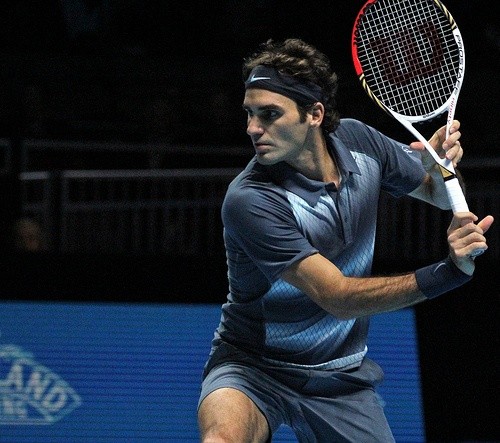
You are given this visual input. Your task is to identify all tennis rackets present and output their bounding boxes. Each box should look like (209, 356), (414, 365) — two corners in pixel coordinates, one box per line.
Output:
(353, 0), (485, 258)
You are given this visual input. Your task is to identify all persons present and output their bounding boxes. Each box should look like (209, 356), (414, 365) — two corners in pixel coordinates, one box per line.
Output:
(198, 42), (494, 443)
(1, 209), (56, 302)
